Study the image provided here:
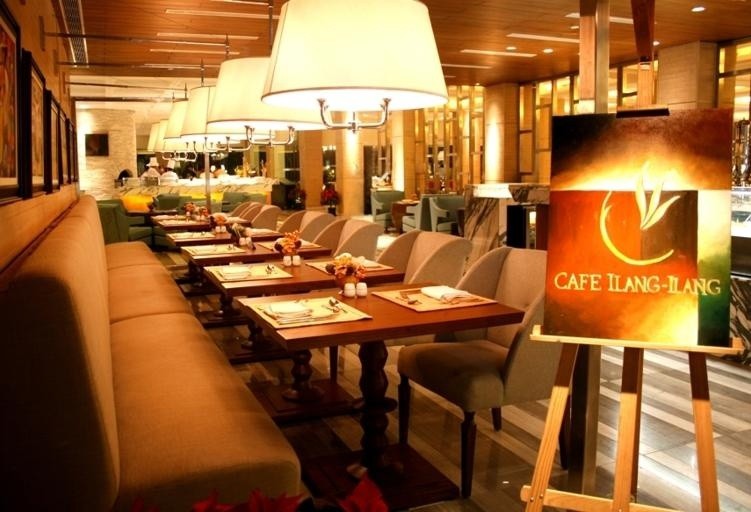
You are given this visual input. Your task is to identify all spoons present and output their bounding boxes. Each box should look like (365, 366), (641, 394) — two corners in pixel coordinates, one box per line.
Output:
(396, 294), (422, 305)
(198, 225), (282, 278)
(323, 294), (350, 314)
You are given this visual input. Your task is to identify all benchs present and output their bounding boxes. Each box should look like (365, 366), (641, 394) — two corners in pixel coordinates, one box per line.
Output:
(0, 194), (303, 512)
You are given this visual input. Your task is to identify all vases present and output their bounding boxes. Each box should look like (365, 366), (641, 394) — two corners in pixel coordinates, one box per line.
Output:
(328, 207), (336, 217)
(294, 203), (305, 211)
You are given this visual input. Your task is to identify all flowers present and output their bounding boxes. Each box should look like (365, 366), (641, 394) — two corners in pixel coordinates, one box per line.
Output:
(320, 183), (340, 208)
(287, 189), (306, 204)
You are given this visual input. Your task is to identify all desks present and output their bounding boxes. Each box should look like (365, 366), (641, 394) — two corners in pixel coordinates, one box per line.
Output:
(391, 201), (420, 234)
(150, 210), (525, 490)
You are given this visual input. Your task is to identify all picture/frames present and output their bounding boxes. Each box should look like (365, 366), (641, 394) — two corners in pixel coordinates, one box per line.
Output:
(0, 0), (78, 207)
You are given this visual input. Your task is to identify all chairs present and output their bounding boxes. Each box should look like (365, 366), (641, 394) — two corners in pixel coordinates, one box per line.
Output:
(429, 196), (465, 234)
(95, 192), (281, 245)
(401, 194), (432, 232)
(398, 246), (563, 495)
(370, 189), (405, 234)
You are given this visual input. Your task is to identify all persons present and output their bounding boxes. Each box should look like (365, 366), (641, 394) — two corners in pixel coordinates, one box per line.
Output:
(112, 157), (236, 186)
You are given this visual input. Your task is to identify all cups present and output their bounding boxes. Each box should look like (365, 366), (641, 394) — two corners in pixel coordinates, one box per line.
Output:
(285, 255), (304, 265)
(238, 236), (251, 247)
(344, 283), (369, 298)
(216, 225), (227, 233)
